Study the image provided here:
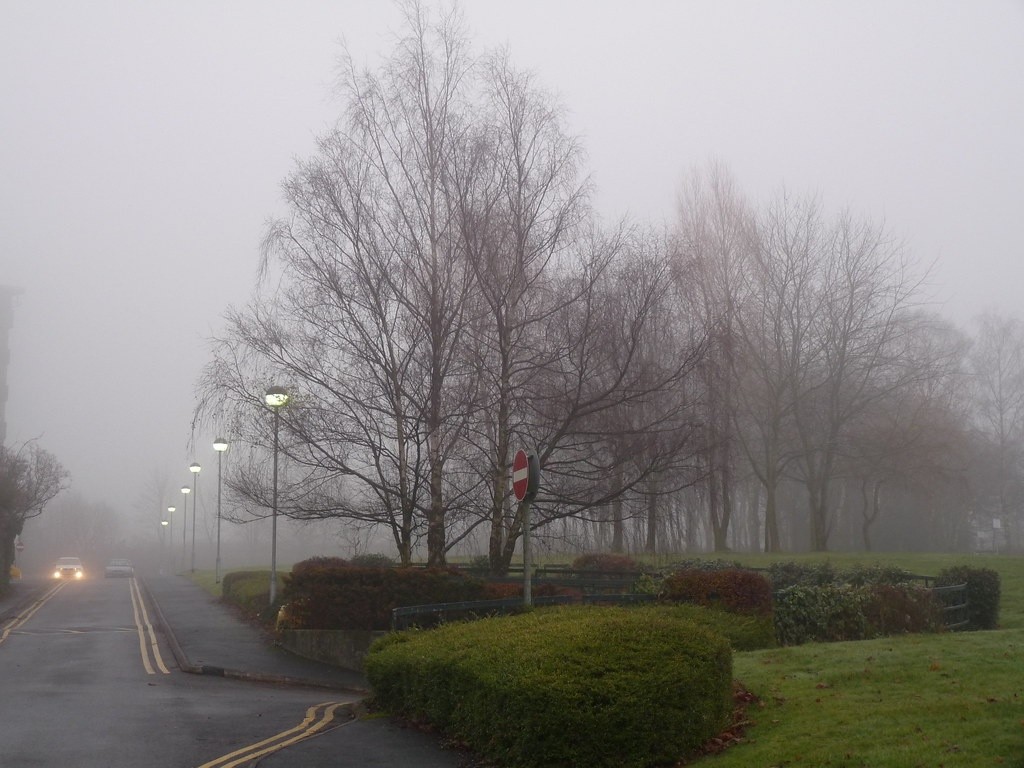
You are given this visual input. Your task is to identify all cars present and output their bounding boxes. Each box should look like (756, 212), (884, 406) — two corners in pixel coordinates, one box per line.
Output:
(55, 557), (83, 578)
(105, 559), (134, 578)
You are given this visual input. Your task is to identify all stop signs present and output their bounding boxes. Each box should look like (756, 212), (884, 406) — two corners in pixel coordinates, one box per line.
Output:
(16, 542), (25, 553)
(512, 448), (528, 501)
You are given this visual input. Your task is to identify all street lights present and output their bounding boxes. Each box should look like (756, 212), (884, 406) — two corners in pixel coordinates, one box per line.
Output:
(190, 463), (201, 572)
(181, 485), (190, 569)
(265, 386), (289, 604)
(168, 506), (176, 564)
(161, 520), (168, 561)
(213, 438), (228, 584)
